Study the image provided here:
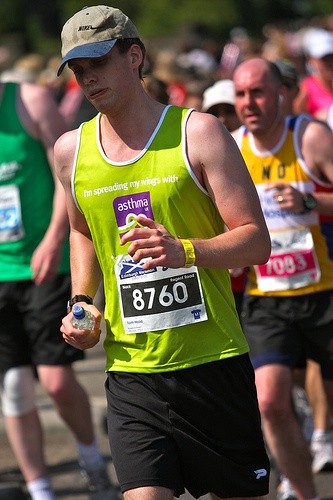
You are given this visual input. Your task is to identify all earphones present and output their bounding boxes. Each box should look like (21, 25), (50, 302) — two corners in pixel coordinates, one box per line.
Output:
(279, 94), (283, 102)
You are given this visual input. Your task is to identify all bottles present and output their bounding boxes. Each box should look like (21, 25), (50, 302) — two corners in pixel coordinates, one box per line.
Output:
(70, 305), (108, 349)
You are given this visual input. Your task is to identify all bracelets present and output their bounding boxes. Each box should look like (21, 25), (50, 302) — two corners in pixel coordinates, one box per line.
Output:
(179, 239), (195, 268)
(67, 295), (93, 311)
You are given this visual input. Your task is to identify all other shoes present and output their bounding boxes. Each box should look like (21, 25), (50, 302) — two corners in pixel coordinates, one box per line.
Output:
(310, 435), (333, 474)
(276, 477), (298, 500)
(78, 460), (116, 500)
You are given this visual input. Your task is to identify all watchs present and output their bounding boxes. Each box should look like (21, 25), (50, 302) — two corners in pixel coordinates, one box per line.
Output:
(299, 195), (315, 215)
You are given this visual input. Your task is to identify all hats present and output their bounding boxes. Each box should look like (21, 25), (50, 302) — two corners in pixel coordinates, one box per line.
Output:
(201, 79), (235, 113)
(272, 60), (298, 82)
(303, 27), (333, 60)
(57, 5), (140, 78)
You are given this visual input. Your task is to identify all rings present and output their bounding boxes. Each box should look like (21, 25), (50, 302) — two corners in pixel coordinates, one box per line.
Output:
(278, 196), (284, 202)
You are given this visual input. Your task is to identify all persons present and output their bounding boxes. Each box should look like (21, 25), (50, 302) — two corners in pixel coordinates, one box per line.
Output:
(55, 5), (272, 500)
(142, 26), (333, 500)
(0, 47), (98, 129)
(0, 81), (117, 500)
